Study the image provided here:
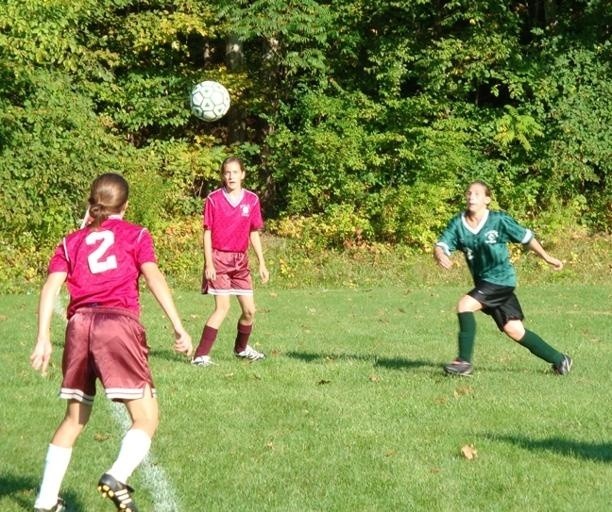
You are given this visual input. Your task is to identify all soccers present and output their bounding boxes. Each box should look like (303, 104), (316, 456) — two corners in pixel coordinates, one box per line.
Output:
(190, 81), (230, 122)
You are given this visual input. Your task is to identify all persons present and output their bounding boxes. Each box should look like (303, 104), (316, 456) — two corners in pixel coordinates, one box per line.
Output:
(30, 172), (195, 510)
(433, 180), (575, 376)
(191, 156), (268, 368)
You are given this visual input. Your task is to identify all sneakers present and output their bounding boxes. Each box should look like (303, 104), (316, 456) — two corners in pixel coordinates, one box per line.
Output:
(232, 343), (265, 360)
(96, 469), (139, 512)
(553, 354), (573, 375)
(191, 355), (217, 367)
(443, 358), (474, 377)
(33, 493), (66, 511)
